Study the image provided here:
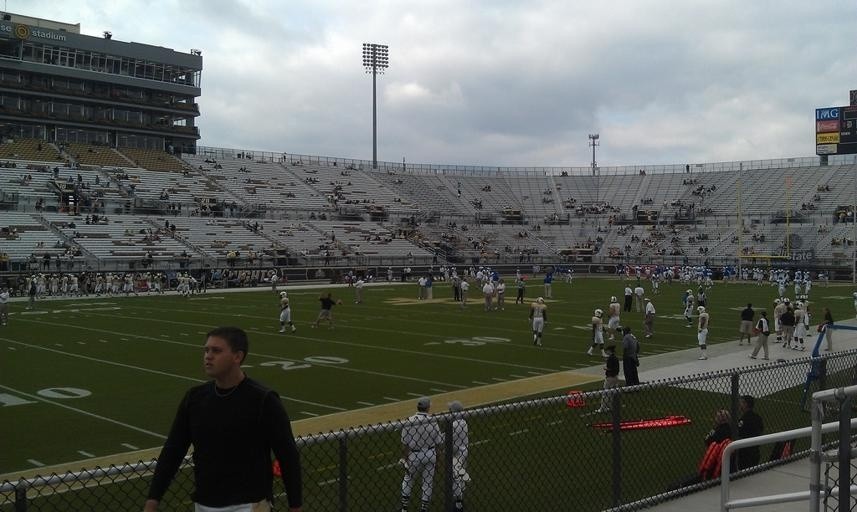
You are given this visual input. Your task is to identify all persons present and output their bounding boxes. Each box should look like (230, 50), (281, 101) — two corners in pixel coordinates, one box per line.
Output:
(399, 397), (445, 512)
(736, 395), (764, 468)
(450, 401), (472, 510)
(705, 411), (731, 445)
(0, 143), (857, 413)
(143, 326), (303, 512)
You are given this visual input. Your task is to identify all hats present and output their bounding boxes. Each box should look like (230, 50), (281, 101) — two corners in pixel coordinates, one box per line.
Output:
(418, 398), (430, 408)
(448, 401), (462, 410)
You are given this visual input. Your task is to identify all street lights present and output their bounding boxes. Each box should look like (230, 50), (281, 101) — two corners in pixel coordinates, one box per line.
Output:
(587, 133), (600, 177)
(361, 42), (390, 171)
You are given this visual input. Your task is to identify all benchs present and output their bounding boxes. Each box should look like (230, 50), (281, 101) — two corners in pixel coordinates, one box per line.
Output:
(0, 130), (856, 296)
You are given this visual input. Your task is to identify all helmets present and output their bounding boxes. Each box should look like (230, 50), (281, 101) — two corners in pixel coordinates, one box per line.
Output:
(595, 309), (604, 317)
(697, 306), (705, 314)
(611, 296), (617, 302)
(686, 289), (693, 295)
(280, 292), (287, 298)
(784, 298), (790, 303)
(774, 299), (781, 306)
(796, 302), (804, 309)
(536, 297), (544, 303)
